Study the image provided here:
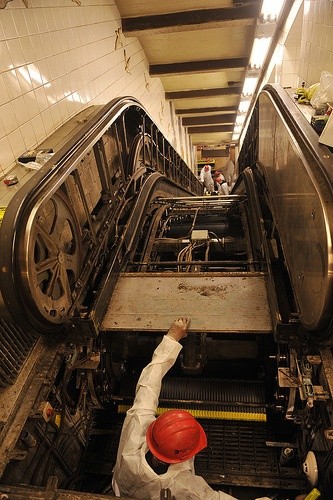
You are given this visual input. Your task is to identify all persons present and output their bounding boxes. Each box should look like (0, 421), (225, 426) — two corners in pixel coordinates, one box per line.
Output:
(111, 316), (311, 500)
(200, 165), (232, 200)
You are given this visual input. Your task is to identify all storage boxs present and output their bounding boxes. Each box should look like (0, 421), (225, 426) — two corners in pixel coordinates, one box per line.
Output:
(18, 148), (54, 163)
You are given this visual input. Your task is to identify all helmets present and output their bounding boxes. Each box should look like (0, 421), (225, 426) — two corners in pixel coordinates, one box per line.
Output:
(214, 171), (220, 176)
(215, 178), (221, 182)
(143, 408), (207, 464)
(205, 165), (210, 171)
